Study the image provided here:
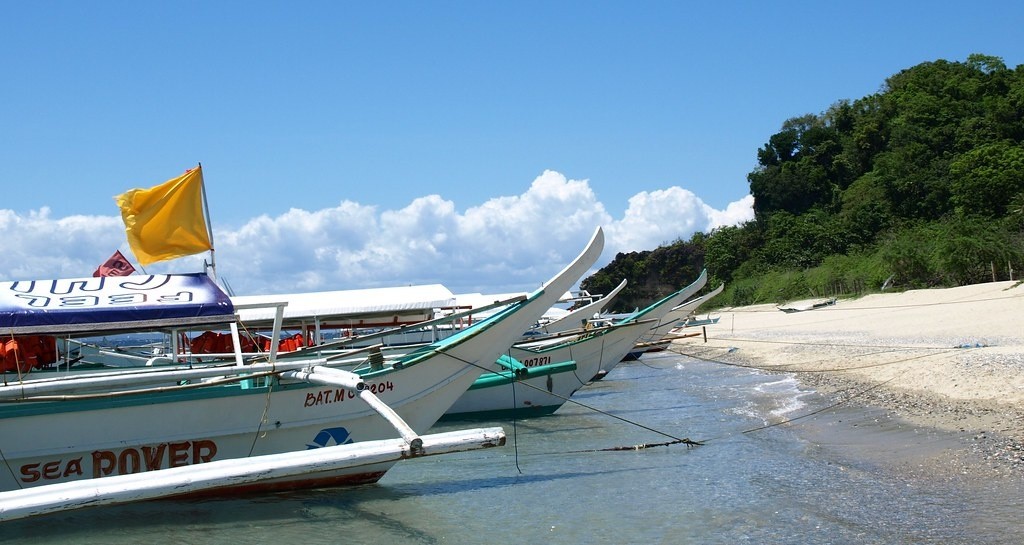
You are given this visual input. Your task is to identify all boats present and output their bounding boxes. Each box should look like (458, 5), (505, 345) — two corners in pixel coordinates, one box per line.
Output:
(775, 297), (838, 314)
(51, 261), (727, 423)
(1, 226), (607, 527)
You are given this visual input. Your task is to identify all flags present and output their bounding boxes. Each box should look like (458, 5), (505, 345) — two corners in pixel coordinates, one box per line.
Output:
(93, 251), (135, 277)
(112, 166), (212, 266)
